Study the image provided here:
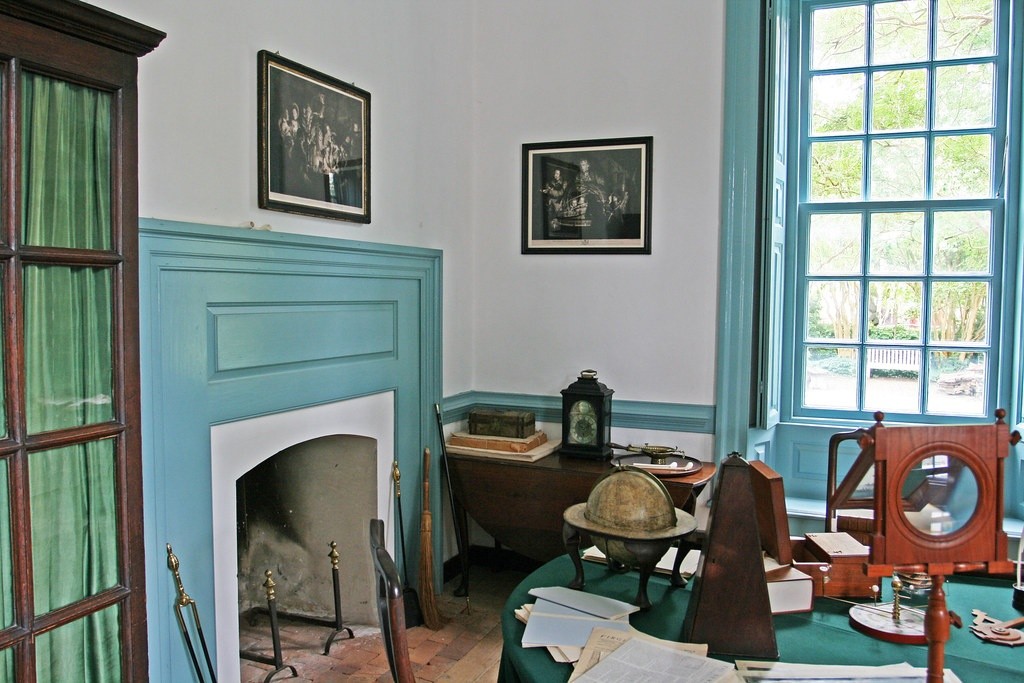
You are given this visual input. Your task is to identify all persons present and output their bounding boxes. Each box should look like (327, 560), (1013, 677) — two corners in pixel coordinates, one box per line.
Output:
(543, 160), (628, 216)
(278, 94), (351, 191)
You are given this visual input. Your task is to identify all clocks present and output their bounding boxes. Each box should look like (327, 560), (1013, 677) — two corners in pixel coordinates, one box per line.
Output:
(557, 370), (615, 464)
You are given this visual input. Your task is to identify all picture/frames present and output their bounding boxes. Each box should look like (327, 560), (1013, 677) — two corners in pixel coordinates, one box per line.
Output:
(256, 49), (372, 224)
(521, 136), (653, 256)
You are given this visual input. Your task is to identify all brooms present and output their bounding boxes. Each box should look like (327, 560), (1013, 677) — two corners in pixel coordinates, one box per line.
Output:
(416, 447), (455, 632)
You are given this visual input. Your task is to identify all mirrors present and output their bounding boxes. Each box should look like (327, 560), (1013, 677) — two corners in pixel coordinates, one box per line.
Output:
(862, 425), (1012, 683)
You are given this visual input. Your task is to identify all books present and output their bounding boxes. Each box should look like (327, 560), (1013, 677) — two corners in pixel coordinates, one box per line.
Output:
(764, 557), (814, 615)
(581, 546), (702, 578)
(514, 587), (641, 663)
(445, 430), (562, 462)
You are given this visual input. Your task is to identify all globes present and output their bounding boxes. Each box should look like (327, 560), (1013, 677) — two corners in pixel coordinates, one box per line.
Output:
(562, 465), (698, 610)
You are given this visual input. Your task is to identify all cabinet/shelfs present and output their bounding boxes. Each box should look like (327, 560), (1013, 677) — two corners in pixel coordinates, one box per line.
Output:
(0, 1), (167, 681)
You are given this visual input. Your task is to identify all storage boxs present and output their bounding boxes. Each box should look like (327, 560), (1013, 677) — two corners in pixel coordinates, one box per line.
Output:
(467, 406), (536, 439)
(748, 459), (879, 598)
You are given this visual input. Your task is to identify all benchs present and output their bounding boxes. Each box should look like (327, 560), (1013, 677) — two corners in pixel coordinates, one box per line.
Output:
(866, 338), (921, 379)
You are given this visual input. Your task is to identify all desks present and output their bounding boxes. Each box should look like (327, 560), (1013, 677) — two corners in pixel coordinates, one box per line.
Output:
(496, 536), (1024, 683)
(440, 449), (717, 598)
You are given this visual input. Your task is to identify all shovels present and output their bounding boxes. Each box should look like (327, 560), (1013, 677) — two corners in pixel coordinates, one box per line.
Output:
(392, 461), (425, 630)
